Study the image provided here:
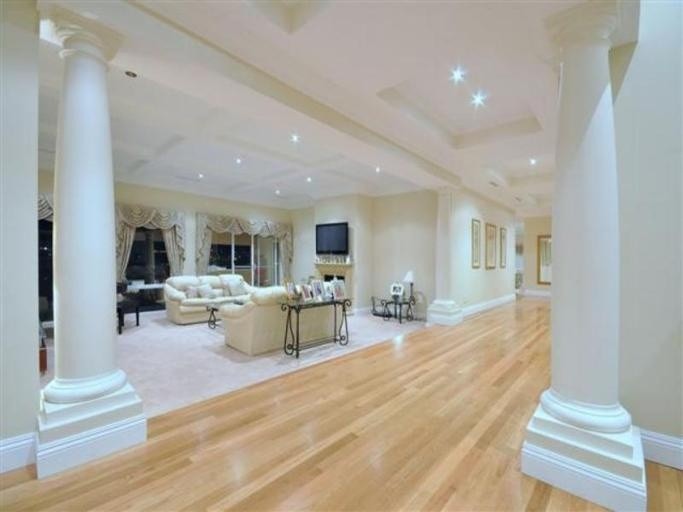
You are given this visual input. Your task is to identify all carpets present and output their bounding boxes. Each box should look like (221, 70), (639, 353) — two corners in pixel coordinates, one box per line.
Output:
(39, 309), (427, 419)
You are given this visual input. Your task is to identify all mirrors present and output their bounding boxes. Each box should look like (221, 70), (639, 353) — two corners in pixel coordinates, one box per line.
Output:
(536, 234), (553, 286)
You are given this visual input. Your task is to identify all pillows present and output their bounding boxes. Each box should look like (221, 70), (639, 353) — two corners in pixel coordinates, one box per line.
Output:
(221, 278), (247, 297)
(185, 283), (217, 299)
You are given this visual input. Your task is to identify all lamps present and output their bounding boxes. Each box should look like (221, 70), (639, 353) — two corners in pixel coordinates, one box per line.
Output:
(403, 271), (416, 301)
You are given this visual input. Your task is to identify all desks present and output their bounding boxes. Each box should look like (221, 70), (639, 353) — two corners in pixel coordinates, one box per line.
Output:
(280, 296), (352, 359)
(370, 295), (416, 324)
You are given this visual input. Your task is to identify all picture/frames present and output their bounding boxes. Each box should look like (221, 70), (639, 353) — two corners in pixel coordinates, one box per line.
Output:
(390, 284), (405, 296)
(471, 218), (507, 270)
(283, 277), (348, 303)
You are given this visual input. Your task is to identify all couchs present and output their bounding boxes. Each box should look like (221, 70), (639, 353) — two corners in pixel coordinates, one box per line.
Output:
(219, 281), (344, 356)
(162, 273), (258, 327)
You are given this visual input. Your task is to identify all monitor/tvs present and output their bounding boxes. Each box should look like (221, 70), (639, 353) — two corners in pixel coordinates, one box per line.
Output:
(316, 223), (348, 254)
